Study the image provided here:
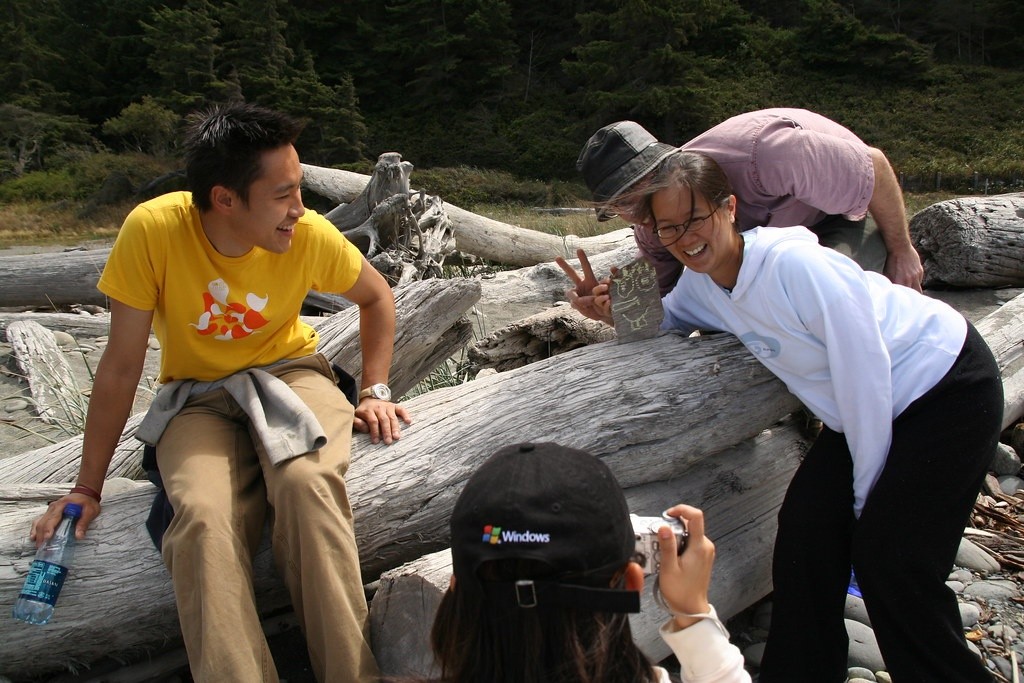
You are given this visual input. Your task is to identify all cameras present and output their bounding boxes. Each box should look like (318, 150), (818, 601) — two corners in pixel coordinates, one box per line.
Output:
(627, 512), (687, 574)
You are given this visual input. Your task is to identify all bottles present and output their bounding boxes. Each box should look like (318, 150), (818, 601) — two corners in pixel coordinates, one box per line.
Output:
(11, 502), (83, 624)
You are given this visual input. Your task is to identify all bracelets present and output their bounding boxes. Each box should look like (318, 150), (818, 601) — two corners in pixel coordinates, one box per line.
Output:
(75, 480), (101, 495)
(68, 486), (102, 503)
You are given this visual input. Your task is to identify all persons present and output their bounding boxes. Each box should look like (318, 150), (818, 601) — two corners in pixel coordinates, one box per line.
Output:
(414, 438), (754, 683)
(30, 102), (413, 680)
(588, 144), (1007, 683)
(556, 100), (925, 315)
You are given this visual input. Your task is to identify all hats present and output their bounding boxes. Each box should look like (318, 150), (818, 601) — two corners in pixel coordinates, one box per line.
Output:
(448, 442), (642, 615)
(576, 120), (681, 221)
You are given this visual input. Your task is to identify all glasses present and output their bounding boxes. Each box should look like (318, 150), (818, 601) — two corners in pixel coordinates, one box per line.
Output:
(650, 203), (722, 240)
(603, 198), (631, 220)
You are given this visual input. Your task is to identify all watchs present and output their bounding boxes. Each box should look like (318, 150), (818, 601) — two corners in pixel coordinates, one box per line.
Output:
(353, 380), (398, 402)
